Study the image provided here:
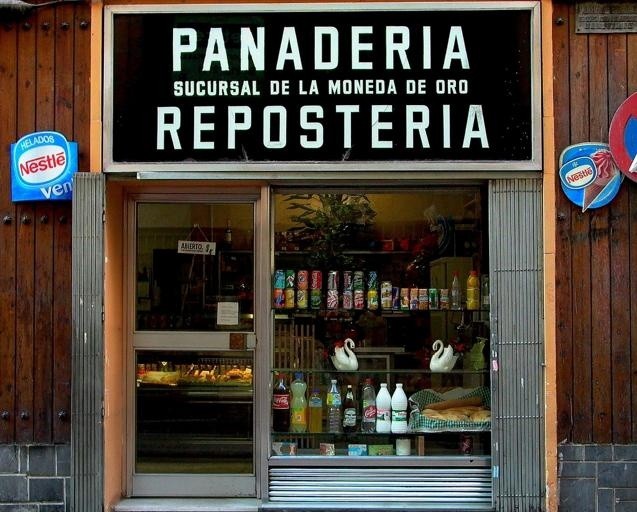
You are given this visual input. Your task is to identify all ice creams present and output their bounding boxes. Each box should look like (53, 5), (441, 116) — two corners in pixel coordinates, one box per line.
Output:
(582, 148), (620, 213)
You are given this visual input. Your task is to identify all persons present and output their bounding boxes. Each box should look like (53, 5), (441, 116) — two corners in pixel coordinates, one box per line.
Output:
(357, 301), (387, 346)
(325, 296), (355, 354)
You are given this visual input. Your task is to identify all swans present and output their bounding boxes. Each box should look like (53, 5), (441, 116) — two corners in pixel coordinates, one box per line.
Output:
(330, 338), (359, 371)
(429, 340), (460, 372)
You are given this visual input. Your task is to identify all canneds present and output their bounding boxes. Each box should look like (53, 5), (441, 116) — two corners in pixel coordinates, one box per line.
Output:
(273, 269), (449, 310)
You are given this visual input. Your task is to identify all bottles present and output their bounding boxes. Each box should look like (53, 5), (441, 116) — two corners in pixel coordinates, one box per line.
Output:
(136, 362), (251, 383)
(448, 268), (489, 309)
(270, 370), (409, 435)
(136, 313), (192, 331)
(222, 216), (233, 246)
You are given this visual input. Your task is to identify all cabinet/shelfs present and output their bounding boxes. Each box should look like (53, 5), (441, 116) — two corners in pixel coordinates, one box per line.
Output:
(270, 185), (493, 467)
(427, 257), (475, 343)
(215, 247), (408, 310)
(137, 310), (290, 434)
(315, 347), (407, 396)
